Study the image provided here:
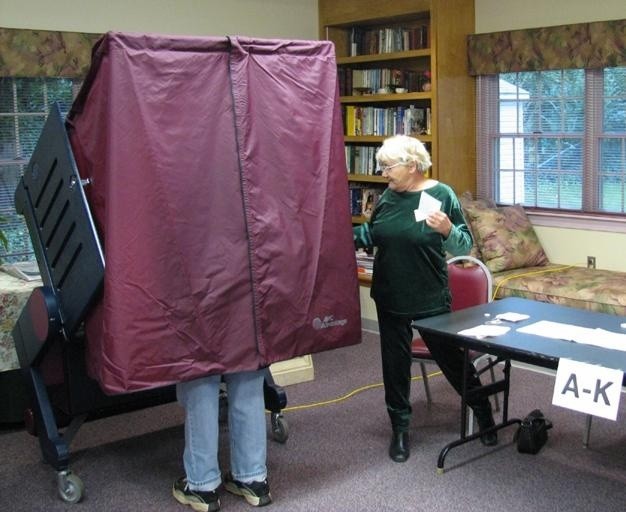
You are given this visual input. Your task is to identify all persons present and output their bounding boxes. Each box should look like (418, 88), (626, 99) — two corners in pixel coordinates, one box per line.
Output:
(172, 366), (272, 511)
(353, 134), (498, 463)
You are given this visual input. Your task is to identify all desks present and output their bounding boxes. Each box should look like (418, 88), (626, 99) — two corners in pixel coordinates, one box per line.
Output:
(410, 296), (626, 474)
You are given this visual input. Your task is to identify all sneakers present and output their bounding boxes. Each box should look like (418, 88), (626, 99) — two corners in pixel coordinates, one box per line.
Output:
(223, 470), (272, 506)
(173, 475), (220, 512)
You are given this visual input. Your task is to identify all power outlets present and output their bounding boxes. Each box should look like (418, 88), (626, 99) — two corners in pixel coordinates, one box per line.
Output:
(587, 256), (596, 268)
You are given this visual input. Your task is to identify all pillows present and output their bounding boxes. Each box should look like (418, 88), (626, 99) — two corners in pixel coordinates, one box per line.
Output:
(446, 190), (549, 273)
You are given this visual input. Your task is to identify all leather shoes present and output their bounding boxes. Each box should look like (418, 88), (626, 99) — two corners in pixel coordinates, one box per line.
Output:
(390, 431), (408, 462)
(480, 418), (497, 446)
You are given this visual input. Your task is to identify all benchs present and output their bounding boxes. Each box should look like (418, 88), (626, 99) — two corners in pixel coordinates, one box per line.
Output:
(454, 264), (626, 316)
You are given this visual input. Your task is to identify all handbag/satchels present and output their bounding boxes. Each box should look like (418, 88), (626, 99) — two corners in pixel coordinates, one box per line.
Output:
(517, 409), (547, 454)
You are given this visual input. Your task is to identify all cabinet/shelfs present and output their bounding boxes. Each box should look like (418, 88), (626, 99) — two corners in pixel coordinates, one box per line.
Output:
(319, 0), (476, 288)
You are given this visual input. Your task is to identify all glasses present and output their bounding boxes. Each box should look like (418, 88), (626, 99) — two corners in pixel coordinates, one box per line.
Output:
(380, 163), (401, 171)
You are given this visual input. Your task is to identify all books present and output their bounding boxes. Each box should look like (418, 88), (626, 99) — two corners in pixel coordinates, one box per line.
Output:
(336, 25), (433, 279)
